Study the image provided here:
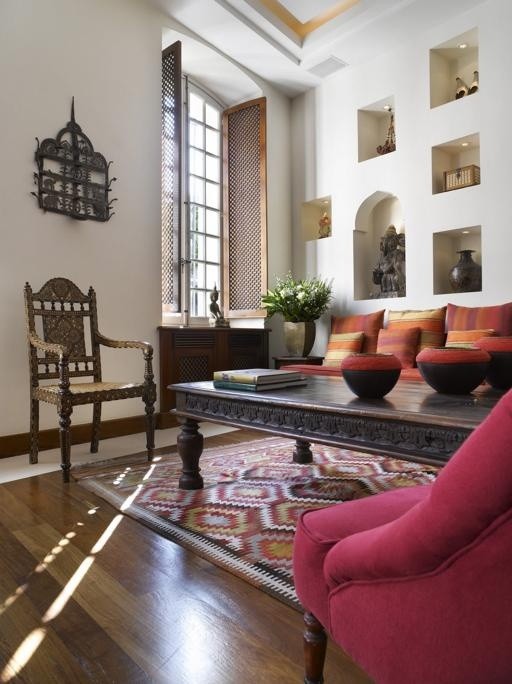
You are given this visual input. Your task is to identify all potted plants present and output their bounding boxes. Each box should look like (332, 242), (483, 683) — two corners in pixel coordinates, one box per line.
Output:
(262, 267), (336, 357)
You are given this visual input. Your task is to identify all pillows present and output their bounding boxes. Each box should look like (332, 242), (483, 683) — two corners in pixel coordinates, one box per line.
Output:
(445, 301), (511, 338)
(323, 330), (365, 367)
(444, 327), (497, 350)
(376, 326), (423, 369)
(331, 309), (390, 354)
(387, 304), (450, 355)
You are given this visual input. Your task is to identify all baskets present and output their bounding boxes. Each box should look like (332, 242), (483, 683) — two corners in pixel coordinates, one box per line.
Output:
(438, 164), (480, 193)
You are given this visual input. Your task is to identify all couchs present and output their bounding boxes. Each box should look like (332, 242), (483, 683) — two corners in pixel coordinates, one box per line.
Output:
(278, 301), (511, 384)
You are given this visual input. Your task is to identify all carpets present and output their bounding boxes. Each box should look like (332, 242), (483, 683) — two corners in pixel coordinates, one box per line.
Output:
(72, 433), (444, 619)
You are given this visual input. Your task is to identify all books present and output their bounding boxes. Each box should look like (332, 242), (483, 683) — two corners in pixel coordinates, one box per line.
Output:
(214, 368), (301, 382)
(215, 379), (306, 393)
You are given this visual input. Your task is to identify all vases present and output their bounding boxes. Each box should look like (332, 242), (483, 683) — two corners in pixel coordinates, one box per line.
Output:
(448, 248), (480, 291)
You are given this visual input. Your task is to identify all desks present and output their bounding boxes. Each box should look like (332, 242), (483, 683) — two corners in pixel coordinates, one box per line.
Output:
(167, 371), (511, 489)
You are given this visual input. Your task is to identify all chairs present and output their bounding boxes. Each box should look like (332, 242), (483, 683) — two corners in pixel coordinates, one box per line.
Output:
(25, 275), (155, 482)
(287, 385), (510, 683)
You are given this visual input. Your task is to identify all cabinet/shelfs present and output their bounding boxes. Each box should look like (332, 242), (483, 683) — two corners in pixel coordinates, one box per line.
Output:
(272, 353), (324, 368)
